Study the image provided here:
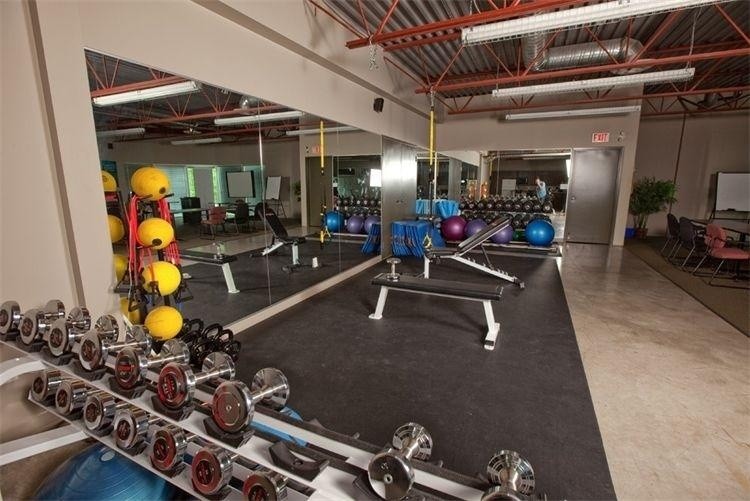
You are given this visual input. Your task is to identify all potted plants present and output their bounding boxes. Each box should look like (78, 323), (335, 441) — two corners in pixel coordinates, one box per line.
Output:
(629, 176), (681, 239)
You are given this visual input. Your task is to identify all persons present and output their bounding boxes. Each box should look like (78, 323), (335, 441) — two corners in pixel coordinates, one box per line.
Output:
(533, 176), (547, 206)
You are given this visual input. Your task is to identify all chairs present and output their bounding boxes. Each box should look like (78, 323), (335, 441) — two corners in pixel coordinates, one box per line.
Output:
(658, 213), (682, 259)
(249, 202), (270, 231)
(691, 223), (749, 284)
(672, 215), (707, 270)
(228, 203), (250, 234)
(198, 206), (232, 239)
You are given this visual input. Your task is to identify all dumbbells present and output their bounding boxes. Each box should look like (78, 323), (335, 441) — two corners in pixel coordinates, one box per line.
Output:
(0, 300), (289, 501)
(458, 199), (552, 229)
(368, 421), (433, 501)
(335, 196), (381, 219)
(481, 449), (536, 501)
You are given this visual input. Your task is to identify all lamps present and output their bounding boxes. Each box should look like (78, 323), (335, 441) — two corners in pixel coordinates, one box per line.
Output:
(507, 105), (641, 122)
(92, 79), (203, 107)
(460, 1), (737, 46)
(491, 65), (695, 98)
(216, 111), (304, 127)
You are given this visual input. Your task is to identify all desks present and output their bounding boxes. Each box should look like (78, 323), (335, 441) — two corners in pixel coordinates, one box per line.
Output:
(163, 205), (247, 241)
(681, 214), (749, 242)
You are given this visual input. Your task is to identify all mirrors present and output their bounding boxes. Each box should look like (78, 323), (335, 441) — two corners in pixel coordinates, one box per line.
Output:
(82, 47), (480, 336)
(485, 150), (575, 248)
